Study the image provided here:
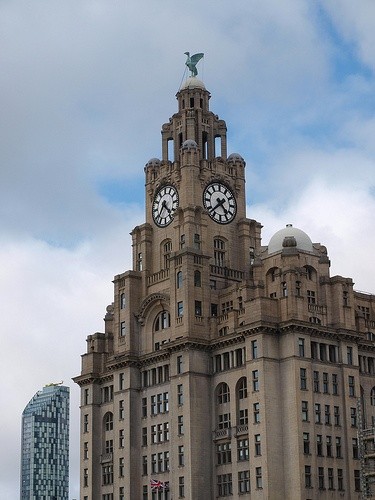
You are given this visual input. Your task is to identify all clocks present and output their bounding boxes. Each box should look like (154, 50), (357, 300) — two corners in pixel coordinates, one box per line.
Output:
(150, 183), (180, 229)
(203, 181), (235, 224)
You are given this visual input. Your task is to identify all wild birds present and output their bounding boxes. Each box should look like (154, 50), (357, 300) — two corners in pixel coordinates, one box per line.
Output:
(184, 52), (204, 77)
